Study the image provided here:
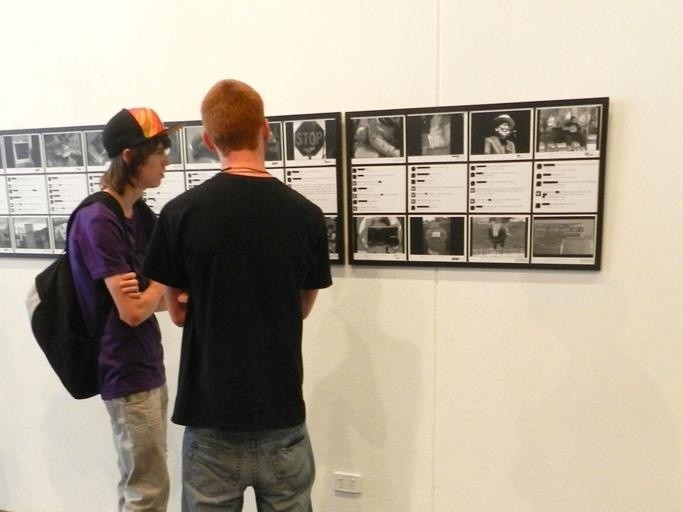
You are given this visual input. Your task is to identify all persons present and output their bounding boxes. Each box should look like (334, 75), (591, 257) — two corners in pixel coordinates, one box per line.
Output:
(66, 105), (188, 512)
(140, 76), (333, 511)
(561, 114), (588, 152)
(482, 114), (517, 153)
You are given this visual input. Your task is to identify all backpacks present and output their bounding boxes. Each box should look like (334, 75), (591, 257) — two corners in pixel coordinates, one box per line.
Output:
(31, 191), (125, 400)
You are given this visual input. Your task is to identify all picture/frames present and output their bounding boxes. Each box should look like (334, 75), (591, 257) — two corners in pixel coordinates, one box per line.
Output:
(344, 95), (611, 273)
(0, 110), (346, 268)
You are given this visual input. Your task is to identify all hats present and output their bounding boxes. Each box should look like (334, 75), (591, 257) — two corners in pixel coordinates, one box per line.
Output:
(101, 107), (188, 160)
(494, 114), (515, 128)
